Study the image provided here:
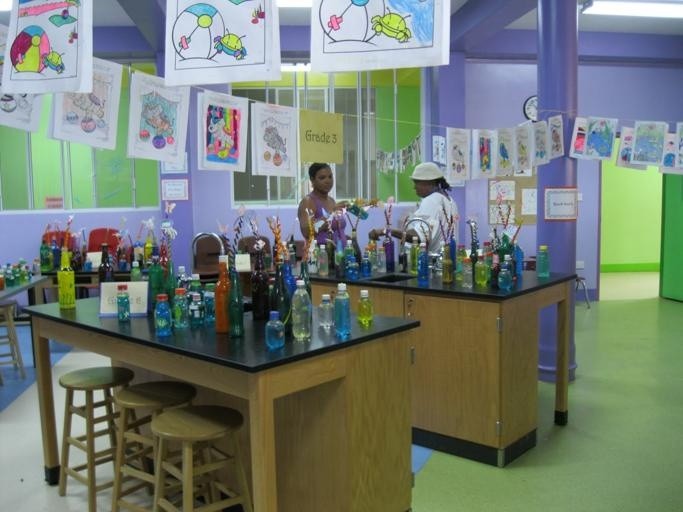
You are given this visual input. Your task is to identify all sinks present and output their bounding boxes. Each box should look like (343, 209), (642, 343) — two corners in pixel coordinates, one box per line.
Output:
(366, 272), (416, 283)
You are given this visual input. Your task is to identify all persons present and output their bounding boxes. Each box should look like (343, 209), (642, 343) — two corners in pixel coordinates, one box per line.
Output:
(297, 162), (350, 245)
(366, 162), (458, 252)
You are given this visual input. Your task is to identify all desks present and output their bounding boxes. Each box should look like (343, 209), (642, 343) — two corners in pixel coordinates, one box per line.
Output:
(308, 269), (578, 468)
(22, 281), (420, 512)
(0, 262), (168, 370)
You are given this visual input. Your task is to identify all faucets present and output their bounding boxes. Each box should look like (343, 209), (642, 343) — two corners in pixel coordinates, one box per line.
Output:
(404, 218), (432, 252)
(191, 231), (226, 255)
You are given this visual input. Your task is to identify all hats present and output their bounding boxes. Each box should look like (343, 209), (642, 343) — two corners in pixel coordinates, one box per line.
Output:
(409, 162), (444, 181)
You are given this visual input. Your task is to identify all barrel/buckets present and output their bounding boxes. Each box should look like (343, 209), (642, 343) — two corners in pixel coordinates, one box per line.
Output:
(42, 230), (74, 249)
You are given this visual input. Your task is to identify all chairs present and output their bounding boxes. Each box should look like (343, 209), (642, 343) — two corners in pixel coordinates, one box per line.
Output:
(41, 229), (72, 256)
(190, 234), (304, 295)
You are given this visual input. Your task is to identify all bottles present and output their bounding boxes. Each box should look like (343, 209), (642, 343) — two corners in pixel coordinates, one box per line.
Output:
(39, 230), (91, 272)
(355, 290), (374, 321)
(0, 257), (31, 290)
(332, 283), (350, 337)
(307, 229), (522, 291)
(116, 283), (131, 323)
(534, 245), (550, 278)
(315, 293), (334, 329)
(56, 245), (76, 310)
(115, 240), (144, 282)
(145, 236), (313, 351)
(98, 243), (113, 282)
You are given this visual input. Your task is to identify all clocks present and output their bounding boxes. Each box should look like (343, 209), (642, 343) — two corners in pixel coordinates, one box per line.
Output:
(523, 95), (537, 123)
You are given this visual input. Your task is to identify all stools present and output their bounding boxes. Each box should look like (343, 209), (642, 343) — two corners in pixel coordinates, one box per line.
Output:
(58, 366), (154, 512)
(112, 381), (210, 512)
(0, 300), (26, 384)
(151, 404), (253, 512)
